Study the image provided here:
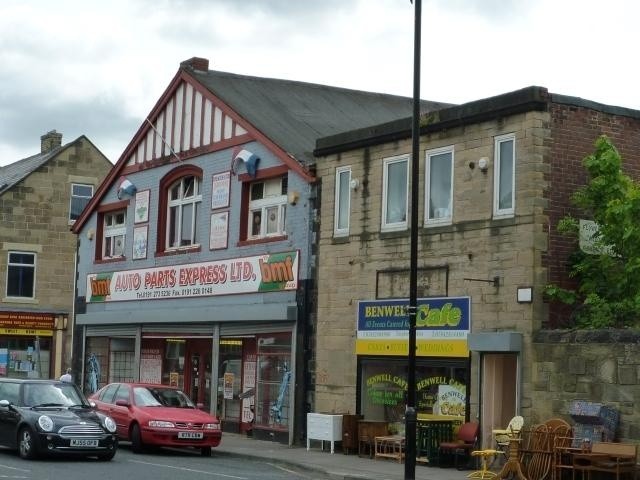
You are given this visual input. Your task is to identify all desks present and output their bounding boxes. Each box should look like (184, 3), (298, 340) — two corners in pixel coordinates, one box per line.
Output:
(415, 418), (456, 467)
(374, 435), (406, 464)
(467, 449), (499, 479)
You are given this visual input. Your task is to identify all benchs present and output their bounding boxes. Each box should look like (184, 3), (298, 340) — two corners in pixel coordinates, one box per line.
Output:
(571, 441), (638, 480)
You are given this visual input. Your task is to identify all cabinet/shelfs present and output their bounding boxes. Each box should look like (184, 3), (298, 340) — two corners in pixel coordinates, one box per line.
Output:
(306, 413), (343, 454)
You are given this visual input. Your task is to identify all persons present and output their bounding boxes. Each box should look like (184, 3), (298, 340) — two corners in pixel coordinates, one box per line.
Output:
(60, 368), (72, 382)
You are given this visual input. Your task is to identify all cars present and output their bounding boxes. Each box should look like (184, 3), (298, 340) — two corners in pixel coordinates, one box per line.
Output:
(0, 376), (118, 462)
(87, 383), (222, 457)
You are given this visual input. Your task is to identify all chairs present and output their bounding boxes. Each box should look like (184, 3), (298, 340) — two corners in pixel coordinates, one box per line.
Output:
(487, 415), (524, 479)
(437, 422), (481, 470)
(510, 418), (575, 480)
